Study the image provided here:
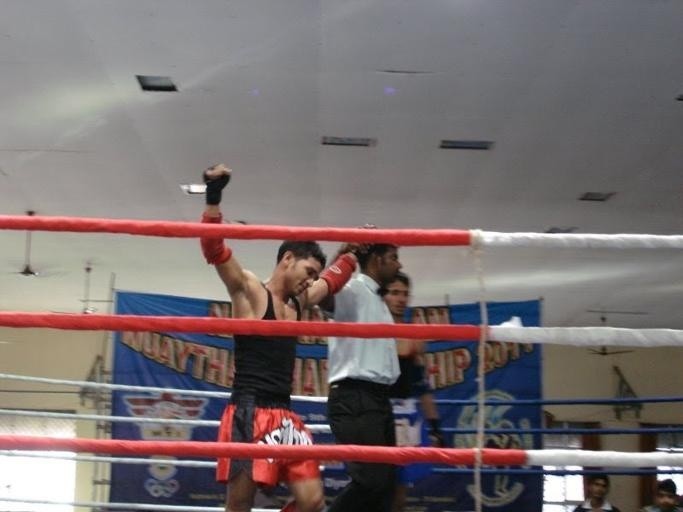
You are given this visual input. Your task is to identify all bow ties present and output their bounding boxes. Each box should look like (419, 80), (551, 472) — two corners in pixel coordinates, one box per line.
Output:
(377, 287), (390, 297)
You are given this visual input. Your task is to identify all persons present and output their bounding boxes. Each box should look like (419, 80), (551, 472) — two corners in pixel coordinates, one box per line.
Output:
(315, 223), (417, 512)
(377, 270), (449, 511)
(643, 478), (681, 512)
(570, 472), (621, 512)
(198, 163), (377, 512)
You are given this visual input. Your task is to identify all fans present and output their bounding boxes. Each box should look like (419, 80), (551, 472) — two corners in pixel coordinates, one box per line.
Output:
(587, 317), (636, 355)
(8, 210), (71, 276)
